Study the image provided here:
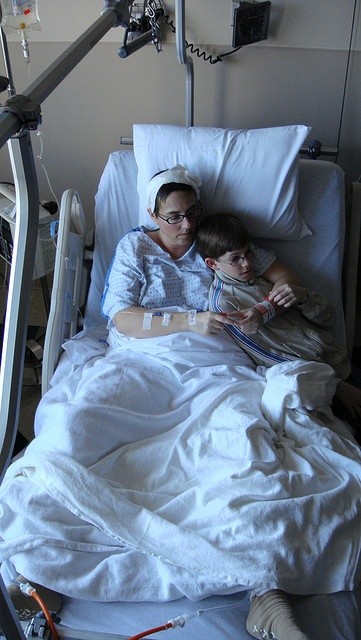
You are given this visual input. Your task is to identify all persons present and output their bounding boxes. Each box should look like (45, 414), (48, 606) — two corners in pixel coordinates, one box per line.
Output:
(197, 211), (361, 433)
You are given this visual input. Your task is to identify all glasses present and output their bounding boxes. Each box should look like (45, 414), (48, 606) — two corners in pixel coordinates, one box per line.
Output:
(156, 198), (202, 224)
(218, 241), (257, 267)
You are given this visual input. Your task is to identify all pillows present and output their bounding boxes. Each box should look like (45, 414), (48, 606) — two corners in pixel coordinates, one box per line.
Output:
(132, 122), (314, 243)
